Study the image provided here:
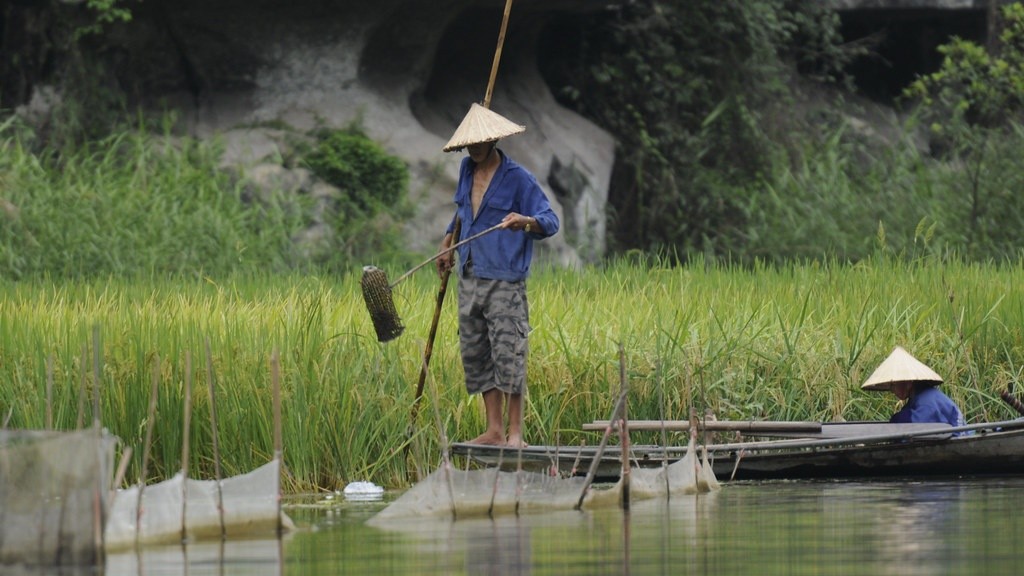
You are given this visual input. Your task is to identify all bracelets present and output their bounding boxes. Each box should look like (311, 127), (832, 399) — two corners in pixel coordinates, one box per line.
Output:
(522, 216), (531, 231)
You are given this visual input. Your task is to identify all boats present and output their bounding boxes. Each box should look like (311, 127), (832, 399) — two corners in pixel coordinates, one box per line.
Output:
(448, 413), (1024, 482)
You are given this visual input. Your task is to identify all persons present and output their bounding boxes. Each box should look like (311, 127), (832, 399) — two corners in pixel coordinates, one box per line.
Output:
(862, 346), (970, 444)
(435, 104), (559, 448)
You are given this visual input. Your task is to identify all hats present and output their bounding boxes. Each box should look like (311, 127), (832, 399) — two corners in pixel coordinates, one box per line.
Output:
(443, 102), (526, 152)
(860, 346), (944, 390)
